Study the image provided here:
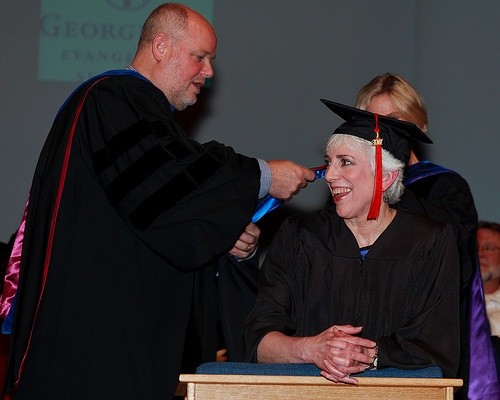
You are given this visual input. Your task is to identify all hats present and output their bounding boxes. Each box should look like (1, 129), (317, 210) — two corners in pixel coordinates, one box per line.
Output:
(320, 99), (433, 220)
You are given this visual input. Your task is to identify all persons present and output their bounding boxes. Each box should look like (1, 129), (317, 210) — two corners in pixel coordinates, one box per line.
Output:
(353, 74), (500, 400)
(478, 221), (500, 385)
(226, 99), (463, 386)
(0, 182), (30, 335)
(6, 2), (316, 400)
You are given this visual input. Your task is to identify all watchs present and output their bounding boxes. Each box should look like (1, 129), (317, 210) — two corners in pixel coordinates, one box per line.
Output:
(367, 345), (379, 370)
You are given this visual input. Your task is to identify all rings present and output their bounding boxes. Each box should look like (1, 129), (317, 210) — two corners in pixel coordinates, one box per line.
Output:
(244, 244), (250, 252)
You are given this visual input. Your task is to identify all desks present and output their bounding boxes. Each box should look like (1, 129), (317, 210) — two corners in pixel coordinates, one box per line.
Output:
(178, 375), (463, 400)
